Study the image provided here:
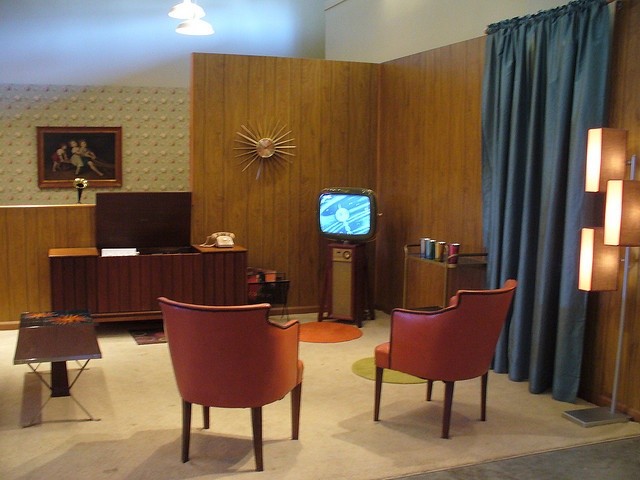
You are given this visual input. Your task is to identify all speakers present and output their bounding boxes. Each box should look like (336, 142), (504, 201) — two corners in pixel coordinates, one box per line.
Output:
(326, 244), (359, 321)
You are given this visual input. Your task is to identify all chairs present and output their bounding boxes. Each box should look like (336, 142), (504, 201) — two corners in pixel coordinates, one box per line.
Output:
(156, 296), (304, 472)
(374, 278), (518, 440)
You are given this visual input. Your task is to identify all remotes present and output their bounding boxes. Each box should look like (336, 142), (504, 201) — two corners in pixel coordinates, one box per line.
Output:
(101, 247), (140, 256)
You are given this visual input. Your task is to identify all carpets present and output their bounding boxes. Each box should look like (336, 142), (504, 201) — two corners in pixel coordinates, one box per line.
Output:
(127, 327), (168, 345)
(351, 357), (429, 384)
(298, 322), (364, 343)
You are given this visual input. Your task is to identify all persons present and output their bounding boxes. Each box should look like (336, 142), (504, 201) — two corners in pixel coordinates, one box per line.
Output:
(51, 143), (66, 172)
(79, 138), (105, 177)
(67, 139), (82, 175)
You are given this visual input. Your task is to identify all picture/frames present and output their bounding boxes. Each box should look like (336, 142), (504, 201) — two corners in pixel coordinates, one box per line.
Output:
(36, 125), (123, 188)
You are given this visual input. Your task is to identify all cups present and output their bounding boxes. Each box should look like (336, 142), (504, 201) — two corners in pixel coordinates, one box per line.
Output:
(425, 239), (436, 260)
(420, 238), (430, 257)
(435, 242), (448, 262)
(447, 243), (460, 263)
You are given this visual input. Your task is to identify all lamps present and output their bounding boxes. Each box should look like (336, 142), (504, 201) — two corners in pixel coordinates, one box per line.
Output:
(168, 0), (216, 36)
(560, 128), (639, 429)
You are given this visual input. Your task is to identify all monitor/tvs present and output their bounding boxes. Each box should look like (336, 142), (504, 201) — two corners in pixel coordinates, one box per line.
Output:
(316, 188), (380, 242)
(95, 191), (193, 254)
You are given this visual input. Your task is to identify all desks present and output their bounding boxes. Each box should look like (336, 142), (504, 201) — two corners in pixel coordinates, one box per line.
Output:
(47, 243), (249, 323)
(403, 243), (489, 310)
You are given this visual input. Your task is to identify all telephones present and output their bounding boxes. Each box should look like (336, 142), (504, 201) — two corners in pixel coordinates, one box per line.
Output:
(199, 231), (235, 248)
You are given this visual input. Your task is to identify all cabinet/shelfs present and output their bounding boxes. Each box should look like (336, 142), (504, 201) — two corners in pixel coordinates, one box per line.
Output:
(317, 244), (376, 327)
